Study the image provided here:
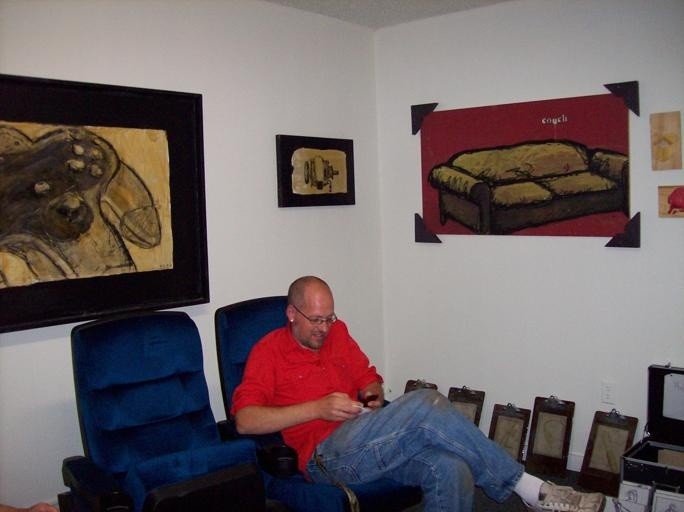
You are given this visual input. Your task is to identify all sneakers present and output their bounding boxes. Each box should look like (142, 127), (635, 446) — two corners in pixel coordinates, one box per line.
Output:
(521, 480), (606, 512)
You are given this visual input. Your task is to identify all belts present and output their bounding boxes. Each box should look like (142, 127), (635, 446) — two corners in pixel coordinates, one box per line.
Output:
(314, 450), (360, 512)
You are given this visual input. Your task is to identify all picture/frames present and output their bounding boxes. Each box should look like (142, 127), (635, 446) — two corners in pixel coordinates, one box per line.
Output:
(2, 72), (209, 334)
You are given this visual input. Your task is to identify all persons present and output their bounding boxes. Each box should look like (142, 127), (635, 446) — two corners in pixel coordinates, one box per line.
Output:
(230, 277), (607, 512)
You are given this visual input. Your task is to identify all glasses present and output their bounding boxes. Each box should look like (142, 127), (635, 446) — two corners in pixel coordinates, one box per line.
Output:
(292, 304), (338, 326)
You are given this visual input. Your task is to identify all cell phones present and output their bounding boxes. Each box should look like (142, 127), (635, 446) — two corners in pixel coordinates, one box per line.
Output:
(360, 393), (379, 407)
